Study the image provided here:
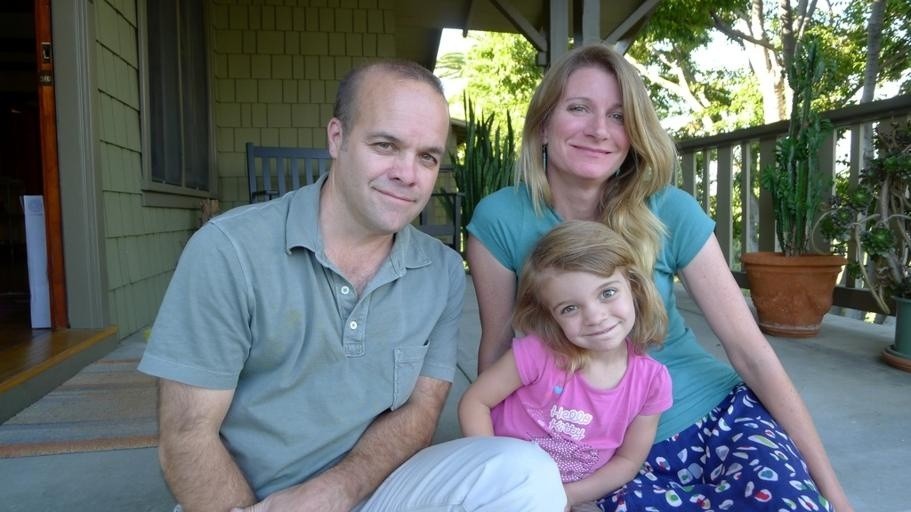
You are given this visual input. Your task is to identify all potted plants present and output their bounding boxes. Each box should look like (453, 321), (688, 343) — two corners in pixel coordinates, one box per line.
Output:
(822, 120), (911, 371)
(739, 24), (866, 339)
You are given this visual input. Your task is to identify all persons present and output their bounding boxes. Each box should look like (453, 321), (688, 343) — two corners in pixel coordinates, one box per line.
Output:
(464, 43), (854, 512)
(136, 58), (568, 512)
(456, 219), (673, 512)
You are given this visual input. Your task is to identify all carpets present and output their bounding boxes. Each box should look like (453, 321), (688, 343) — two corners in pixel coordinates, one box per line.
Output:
(0, 358), (159, 458)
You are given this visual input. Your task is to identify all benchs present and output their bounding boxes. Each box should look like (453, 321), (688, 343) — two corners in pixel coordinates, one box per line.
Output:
(246, 143), (465, 252)
